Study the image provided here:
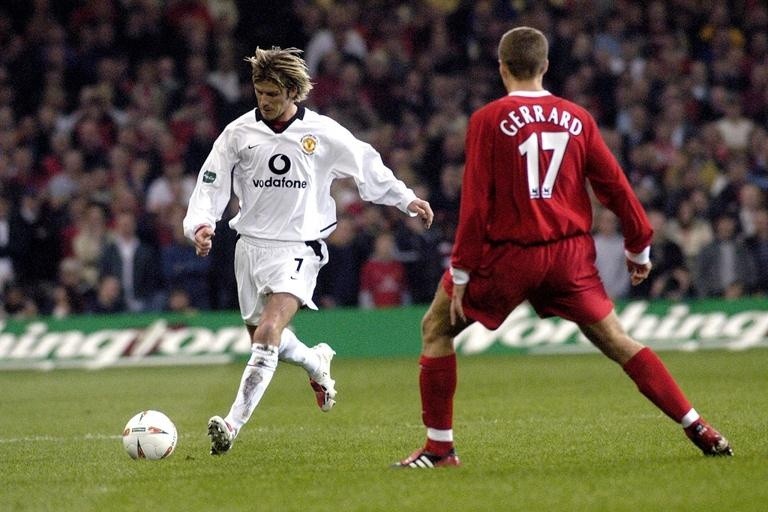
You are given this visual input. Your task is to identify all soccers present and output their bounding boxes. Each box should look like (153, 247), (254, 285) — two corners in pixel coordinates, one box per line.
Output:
(123, 410), (177, 461)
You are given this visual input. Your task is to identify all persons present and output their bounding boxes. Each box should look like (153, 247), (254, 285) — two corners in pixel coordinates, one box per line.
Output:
(1, 1), (767, 309)
(181, 45), (434, 455)
(392, 26), (734, 470)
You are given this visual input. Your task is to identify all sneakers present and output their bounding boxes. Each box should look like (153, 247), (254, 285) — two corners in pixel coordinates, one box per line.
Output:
(308, 342), (337, 413)
(393, 448), (460, 469)
(208, 415), (238, 454)
(683, 416), (733, 457)
